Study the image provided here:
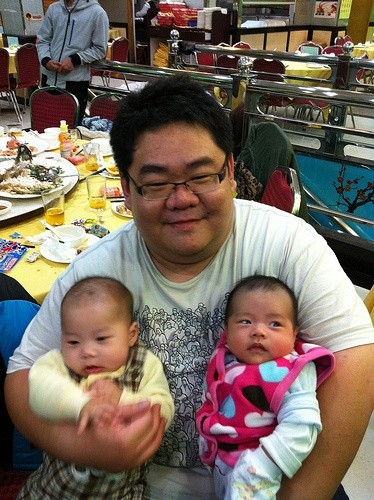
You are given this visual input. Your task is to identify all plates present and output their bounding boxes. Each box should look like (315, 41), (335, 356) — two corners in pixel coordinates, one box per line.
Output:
(111, 201), (135, 218)
(86, 138), (113, 156)
(39, 234), (102, 264)
(0, 136), (49, 159)
(228, 41), (374, 68)
(39, 133), (61, 150)
(99, 164), (122, 180)
(0, 157), (72, 198)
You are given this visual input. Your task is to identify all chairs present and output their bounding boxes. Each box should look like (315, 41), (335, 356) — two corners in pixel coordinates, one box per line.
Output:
(0, 37), (130, 134)
(193, 35), (364, 128)
(259, 165), (301, 217)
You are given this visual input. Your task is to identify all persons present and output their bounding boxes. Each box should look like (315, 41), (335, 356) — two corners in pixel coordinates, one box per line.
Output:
(18, 276), (175, 499)
(135, 0), (159, 45)
(196, 275), (337, 500)
(0, 273), (44, 500)
(5, 73), (374, 500)
(36, 0), (110, 129)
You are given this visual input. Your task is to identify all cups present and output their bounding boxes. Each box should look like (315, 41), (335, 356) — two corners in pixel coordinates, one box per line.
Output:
(86, 175), (107, 211)
(41, 188), (65, 226)
(7, 124), (23, 137)
(196, 6), (227, 40)
(83, 143), (101, 171)
(52, 223), (85, 245)
(0, 126), (4, 137)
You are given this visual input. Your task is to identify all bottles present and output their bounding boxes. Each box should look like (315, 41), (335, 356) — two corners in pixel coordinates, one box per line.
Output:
(58, 121), (73, 160)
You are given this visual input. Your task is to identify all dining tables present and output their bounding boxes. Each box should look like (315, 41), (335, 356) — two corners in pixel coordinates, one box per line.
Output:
(351, 43), (374, 61)
(0, 134), (134, 308)
(241, 58), (332, 126)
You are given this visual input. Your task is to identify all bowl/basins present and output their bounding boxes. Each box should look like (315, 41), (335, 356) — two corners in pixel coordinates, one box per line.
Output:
(44, 127), (60, 136)
(0, 200), (12, 215)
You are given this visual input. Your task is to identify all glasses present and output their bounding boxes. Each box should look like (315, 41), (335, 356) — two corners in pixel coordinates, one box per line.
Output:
(126, 155), (228, 201)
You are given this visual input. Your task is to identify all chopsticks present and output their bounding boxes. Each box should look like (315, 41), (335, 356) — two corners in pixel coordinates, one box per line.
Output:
(79, 168), (106, 183)
(76, 143), (90, 156)
(107, 196), (125, 202)
